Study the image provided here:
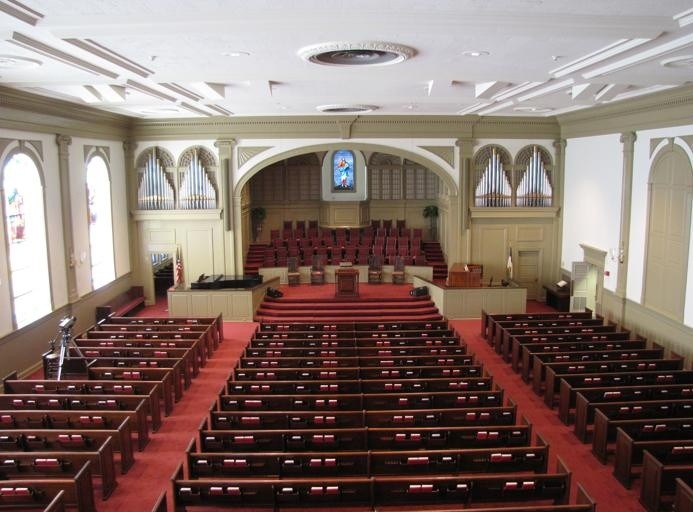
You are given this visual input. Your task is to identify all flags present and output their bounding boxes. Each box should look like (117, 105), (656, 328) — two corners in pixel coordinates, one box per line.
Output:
(506, 247), (513, 280)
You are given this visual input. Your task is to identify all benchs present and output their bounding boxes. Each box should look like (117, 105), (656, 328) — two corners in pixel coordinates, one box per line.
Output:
(0, 287), (693, 512)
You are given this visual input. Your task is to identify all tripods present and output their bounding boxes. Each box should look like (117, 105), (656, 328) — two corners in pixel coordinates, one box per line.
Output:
(57, 333), (89, 381)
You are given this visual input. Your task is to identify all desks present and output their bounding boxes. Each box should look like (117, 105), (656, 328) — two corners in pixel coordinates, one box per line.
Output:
(544, 282), (570, 310)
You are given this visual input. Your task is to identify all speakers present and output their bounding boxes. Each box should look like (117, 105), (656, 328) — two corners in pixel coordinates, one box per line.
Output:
(267, 287), (283, 297)
(409, 286), (427, 296)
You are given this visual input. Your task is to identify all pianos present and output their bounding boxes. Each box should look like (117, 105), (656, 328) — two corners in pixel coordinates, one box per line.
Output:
(189, 274), (263, 290)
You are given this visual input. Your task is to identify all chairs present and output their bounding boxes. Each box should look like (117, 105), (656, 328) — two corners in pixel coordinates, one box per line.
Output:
(263, 220), (426, 285)
